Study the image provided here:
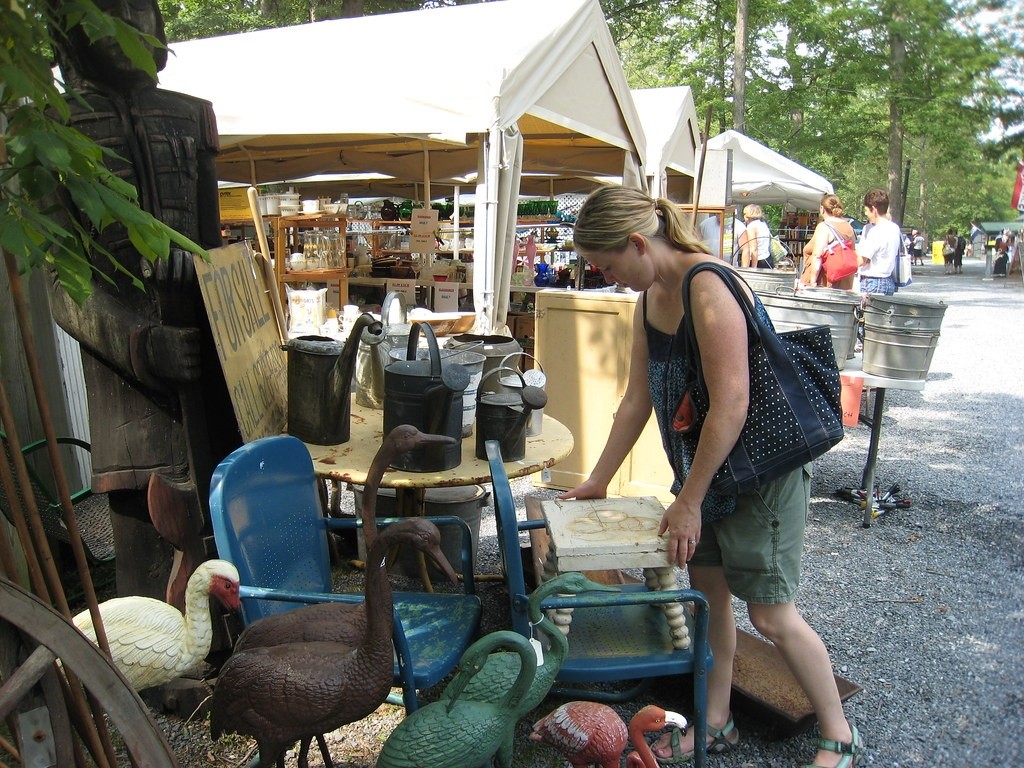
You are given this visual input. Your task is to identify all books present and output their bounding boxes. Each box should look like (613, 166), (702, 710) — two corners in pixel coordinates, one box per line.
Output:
(778, 224), (817, 279)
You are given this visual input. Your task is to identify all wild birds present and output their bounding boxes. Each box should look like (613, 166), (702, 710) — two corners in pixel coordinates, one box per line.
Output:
(374, 570), (623, 768)
(207, 515), (461, 768)
(227, 419), (461, 768)
(52, 558), (243, 707)
(528, 700), (688, 768)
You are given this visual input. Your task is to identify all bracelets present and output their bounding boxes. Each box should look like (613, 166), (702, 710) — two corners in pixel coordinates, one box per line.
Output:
(810, 280), (817, 285)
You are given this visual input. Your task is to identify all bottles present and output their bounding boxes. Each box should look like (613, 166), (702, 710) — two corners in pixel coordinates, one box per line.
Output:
(534, 263), (550, 287)
(381, 198), (454, 221)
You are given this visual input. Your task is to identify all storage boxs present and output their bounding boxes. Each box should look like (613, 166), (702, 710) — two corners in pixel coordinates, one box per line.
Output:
(218, 186), (262, 219)
(503, 309), (539, 371)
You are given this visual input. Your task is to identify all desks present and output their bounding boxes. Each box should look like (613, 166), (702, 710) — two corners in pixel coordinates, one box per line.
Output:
(277, 275), (566, 316)
(816, 355), (926, 528)
(294, 410), (575, 588)
(338, 242), (559, 272)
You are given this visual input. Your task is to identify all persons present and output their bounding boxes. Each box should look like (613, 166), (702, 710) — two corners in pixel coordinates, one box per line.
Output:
(558, 184), (866, 768)
(942, 227), (966, 275)
(346, 233), (357, 253)
(994, 227), (1024, 278)
(854, 188), (913, 296)
(902, 230), (925, 267)
(800, 193), (859, 290)
(698, 204), (788, 270)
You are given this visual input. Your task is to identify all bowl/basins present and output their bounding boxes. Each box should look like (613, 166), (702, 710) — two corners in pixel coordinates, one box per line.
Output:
(433, 274), (447, 282)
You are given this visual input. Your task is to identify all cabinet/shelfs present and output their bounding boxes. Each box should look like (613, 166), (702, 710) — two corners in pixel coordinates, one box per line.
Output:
(215, 214), (350, 313)
(533, 284), (679, 505)
(780, 238), (811, 256)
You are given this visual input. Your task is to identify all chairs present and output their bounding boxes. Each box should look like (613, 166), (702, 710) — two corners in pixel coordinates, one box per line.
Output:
(210, 430), (465, 768)
(486, 428), (717, 768)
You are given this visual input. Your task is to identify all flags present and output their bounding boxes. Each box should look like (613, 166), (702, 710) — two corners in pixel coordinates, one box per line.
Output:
(1011, 161), (1024, 212)
(970, 221), (981, 244)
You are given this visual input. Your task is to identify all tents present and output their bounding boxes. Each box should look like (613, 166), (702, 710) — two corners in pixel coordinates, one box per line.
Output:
(697, 130), (834, 214)
(20, 0), (701, 336)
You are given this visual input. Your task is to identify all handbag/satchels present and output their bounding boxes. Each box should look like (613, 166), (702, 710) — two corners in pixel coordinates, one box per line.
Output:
(769, 235), (787, 261)
(668, 262), (845, 496)
(943, 245), (955, 255)
(891, 232), (912, 286)
(821, 221), (858, 281)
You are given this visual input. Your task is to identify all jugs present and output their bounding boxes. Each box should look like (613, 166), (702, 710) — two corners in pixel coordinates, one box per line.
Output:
(497, 352), (547, 438)
(354, 291), (411, 410)
(474, 367), (548, 463)
(382, 321), (471, 473)
(279, 313), (383, 447)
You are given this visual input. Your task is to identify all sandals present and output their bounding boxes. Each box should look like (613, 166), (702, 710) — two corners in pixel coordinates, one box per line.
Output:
(650, 710), (739, 763)
(809, 723), (863, 768)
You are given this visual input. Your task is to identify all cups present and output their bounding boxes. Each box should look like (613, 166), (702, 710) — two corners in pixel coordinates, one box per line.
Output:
(284, 229), (346, 271)
(517, 200), (558, 218)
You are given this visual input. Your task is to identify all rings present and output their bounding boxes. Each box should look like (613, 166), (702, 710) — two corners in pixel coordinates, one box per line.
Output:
(689, 540), (696, 544)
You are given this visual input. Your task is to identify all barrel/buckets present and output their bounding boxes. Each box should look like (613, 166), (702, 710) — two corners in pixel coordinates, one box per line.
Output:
(387, 340), (487, 439)
(729, 237), (948, 382)
(441, 332), (523, 396)
